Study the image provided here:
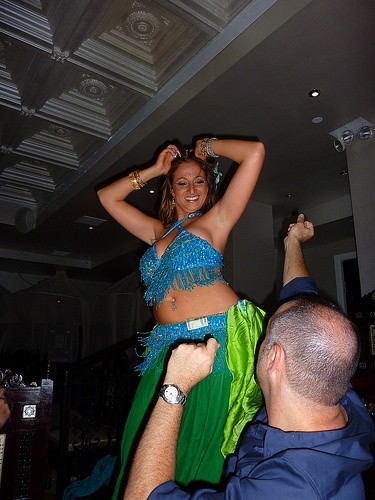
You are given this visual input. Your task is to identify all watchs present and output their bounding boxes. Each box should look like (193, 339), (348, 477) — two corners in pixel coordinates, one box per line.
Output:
(158, 384), (187, 405)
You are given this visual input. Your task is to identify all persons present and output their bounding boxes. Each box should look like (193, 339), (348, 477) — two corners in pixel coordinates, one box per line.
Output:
(97, 137), (269, 500)
(120, 214), (375, 500)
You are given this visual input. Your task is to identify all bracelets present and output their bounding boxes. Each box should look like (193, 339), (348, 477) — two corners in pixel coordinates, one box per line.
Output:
(129, 169), (147, 190)
(200, 137), (219, 158)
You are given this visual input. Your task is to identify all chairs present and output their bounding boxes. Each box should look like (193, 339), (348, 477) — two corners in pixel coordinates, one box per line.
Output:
(0, 370), (58, 500)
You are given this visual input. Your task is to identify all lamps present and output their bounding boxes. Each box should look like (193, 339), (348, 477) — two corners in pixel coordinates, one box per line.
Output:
(333, 125), (373, 153)
